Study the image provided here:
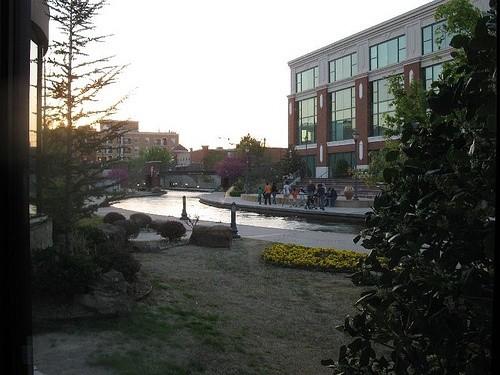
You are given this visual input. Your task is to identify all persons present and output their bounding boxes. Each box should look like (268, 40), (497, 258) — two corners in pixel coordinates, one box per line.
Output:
(281, 181), (298, 208)
(262, 180), (272, 205)
(256, 187), (264, 205)
(326, 187), (338, 206)
(317, 184), (326, 211)
(305, 180), (318, 210)
(271, 183), (279, 204)
(290, 187), (298, 207)
(298, 186), (305, 206)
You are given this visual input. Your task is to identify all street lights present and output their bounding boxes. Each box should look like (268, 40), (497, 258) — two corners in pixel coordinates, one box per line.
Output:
(351, 134), (360, 201)
